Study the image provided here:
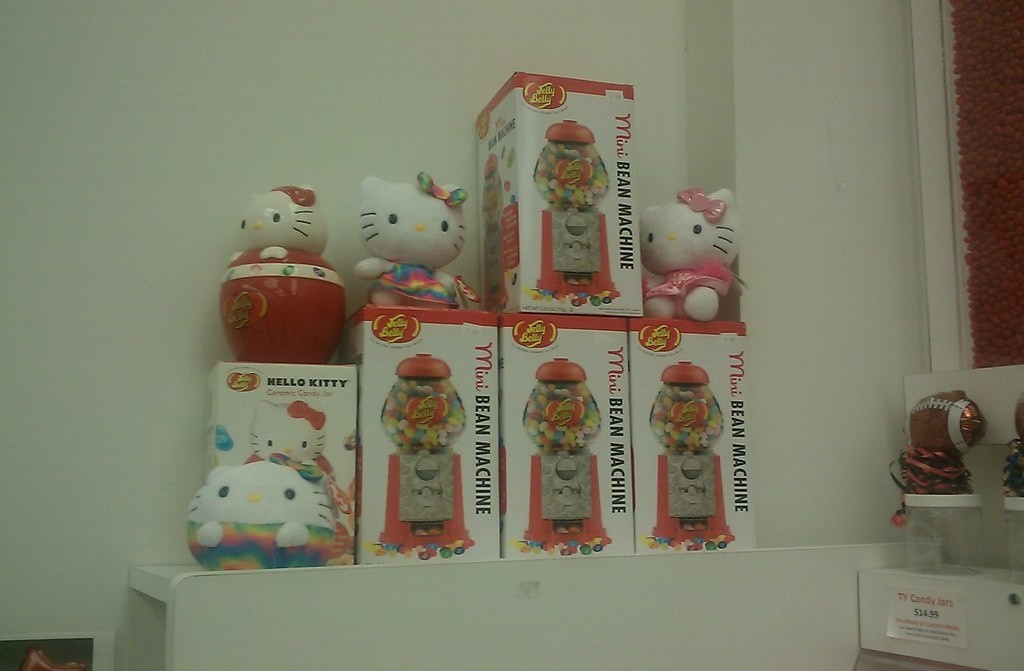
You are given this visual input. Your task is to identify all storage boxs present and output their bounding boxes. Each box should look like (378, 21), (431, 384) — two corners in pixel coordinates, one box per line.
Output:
(476, 71), (644, 318)
(204, 362), (357, 566)
(334, 303), (757, 566)
